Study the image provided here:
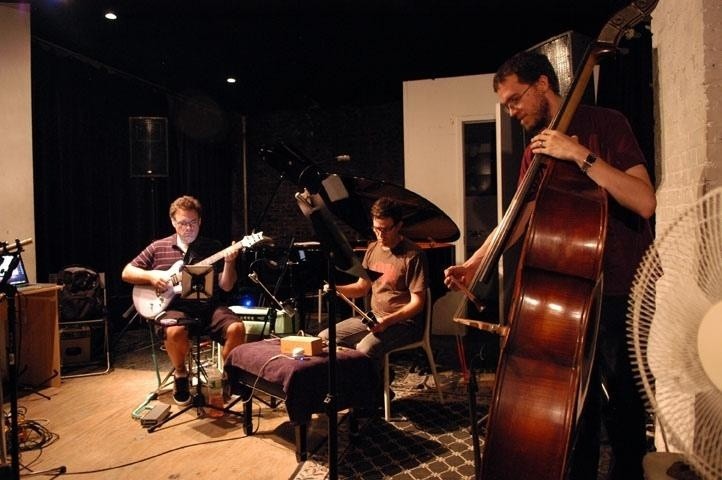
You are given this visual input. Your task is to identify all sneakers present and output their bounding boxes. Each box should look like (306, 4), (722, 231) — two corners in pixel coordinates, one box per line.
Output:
(172, 372), (191, 405)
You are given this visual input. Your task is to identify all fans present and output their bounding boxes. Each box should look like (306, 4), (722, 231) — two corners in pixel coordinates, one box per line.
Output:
(624, 184), (721, 480)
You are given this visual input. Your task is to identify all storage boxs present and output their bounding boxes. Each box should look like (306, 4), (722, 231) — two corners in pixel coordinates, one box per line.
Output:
(229, 305), (299, 338)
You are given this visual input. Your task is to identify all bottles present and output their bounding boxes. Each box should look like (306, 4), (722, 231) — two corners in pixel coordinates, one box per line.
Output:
(207, 377), (224, 417)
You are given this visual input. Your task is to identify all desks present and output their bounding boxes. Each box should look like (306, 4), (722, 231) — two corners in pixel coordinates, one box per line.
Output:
(1, 283), (66, 412)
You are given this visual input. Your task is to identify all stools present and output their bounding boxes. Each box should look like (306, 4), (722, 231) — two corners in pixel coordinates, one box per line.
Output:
(232, 336), (373, 462)
(151, 334), (213, 400)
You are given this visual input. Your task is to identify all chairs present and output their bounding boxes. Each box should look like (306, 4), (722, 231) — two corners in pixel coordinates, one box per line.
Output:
(353, 287), (445, 421)
(48, 268), (110, 378)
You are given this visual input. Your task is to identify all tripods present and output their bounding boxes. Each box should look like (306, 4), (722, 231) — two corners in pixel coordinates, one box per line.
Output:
(147, 266), (241, 433)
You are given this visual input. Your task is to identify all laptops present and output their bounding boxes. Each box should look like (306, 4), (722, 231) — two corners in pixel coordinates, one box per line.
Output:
(0, 252), (40, 292)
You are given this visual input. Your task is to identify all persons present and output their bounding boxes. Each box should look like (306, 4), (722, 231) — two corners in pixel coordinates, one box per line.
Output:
(318, 197), (428, 421)
(121, 196), (246, 407)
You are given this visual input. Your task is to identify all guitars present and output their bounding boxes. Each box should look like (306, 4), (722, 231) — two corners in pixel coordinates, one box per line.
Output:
(131, 230), (266, 319)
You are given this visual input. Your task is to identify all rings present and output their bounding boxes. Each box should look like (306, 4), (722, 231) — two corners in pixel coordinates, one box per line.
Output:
(540, 140), (544, 148)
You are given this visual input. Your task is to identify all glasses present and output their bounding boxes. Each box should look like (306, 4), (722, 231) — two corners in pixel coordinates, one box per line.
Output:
(502, 84), (531, 109)
(173, 216), (199, 226)
(372, 224), (395, 232)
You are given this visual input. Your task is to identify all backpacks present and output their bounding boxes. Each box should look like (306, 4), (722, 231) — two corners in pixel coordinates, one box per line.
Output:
(57, 266), (106, 322)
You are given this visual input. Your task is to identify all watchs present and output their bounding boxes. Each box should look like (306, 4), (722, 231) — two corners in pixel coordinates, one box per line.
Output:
(581, 151), (598, 174)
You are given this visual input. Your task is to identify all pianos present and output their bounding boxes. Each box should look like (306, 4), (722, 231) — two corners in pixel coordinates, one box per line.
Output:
(252, 172), (460, 331)
